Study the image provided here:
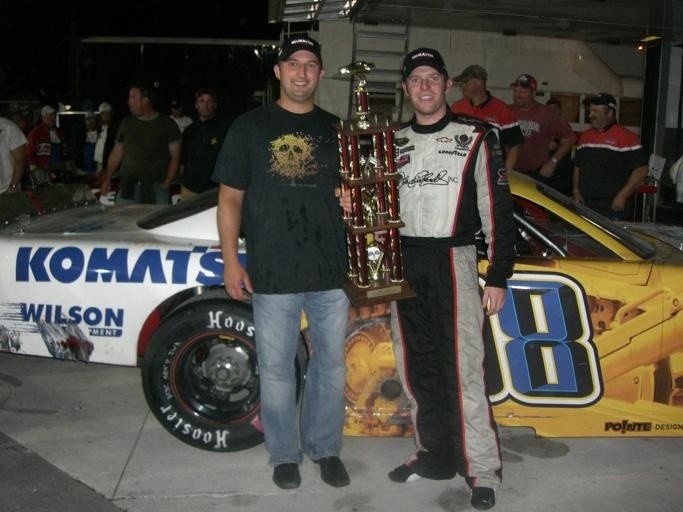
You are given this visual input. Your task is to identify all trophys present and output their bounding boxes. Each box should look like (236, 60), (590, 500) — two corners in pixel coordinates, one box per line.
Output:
(331, 60), (418, 309)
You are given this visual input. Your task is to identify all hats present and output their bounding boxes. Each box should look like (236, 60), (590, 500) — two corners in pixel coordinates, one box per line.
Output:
(275, 34), (322, 66)
(85, 103), (111, 118)
(510, 74), (538, 91)
(194, 87), (215, 98)
(402, 47), (449, 80)
(582, 93), (618, 110)
(40, 105), (56, 116)
(454, 64), (487, 83)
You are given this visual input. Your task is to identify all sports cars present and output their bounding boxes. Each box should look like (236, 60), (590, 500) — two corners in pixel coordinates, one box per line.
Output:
(0, 167), (682, 452)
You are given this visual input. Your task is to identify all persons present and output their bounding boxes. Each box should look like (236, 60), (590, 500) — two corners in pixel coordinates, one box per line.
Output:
(450, 64), (527, 169)
(570, 92), (649, 221)
(0, 81), (233, 206)
(507, 73), (579, 181)
(545, 98), (561, 115)
(386, 46), (518, 510)
(209, 34), (360, 489)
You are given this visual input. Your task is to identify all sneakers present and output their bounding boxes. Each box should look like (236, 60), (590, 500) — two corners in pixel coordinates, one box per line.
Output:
(471, 485), (495, 508)
(273, 462), (301, 488)
(388, 463), (423, 483)
(314, 454), (349, 487)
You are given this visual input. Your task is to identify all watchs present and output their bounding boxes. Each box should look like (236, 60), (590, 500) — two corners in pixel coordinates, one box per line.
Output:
(549, 155), (561, 171)
